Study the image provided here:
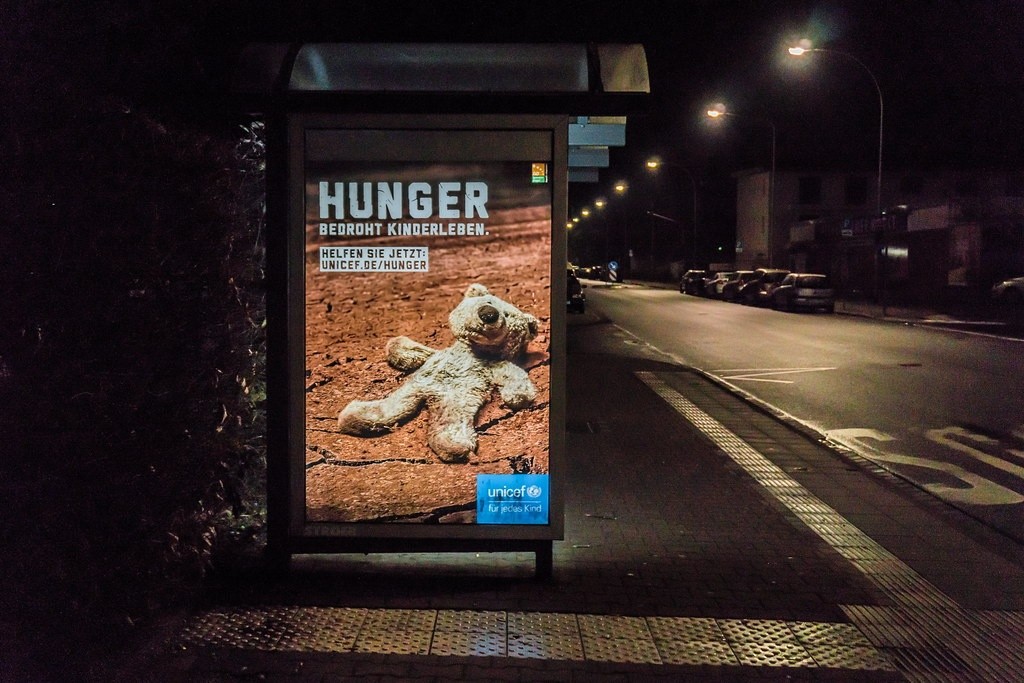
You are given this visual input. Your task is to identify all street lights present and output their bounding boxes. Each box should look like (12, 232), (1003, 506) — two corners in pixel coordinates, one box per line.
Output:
(788, 45), (886, 316)
(707, 103), (776, 268)
(648, 162), (697, 256)
(616, 185), (632, 279)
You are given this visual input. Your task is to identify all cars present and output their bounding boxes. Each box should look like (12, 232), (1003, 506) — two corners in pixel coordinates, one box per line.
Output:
(991, 277), (1024, 330)
(734, 268), (791, 308)
(567, 269), (576, 279)
(567, 275), (587, 314)
(768, 273), (838, 314)
(679, 270), (714, 297)
(722, 271), (754, 300)
(703, 273), (734, 298)
(575, 266), (623, 283)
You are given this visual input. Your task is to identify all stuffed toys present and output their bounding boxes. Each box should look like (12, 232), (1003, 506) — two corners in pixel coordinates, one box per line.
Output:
(338, 284), (539, 461)
